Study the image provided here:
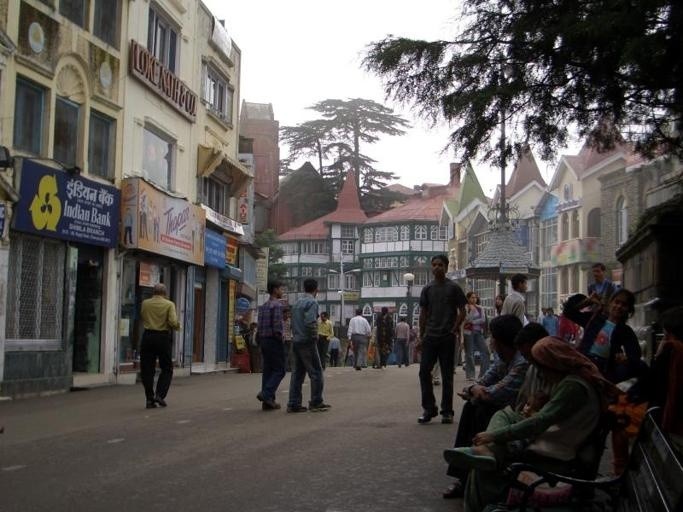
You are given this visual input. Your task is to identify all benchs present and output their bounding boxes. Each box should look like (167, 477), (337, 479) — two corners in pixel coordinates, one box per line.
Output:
(500, 376), (683, 512)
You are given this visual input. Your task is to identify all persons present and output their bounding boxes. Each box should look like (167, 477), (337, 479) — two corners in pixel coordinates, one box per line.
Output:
(123, 206), (133, 247)
(138, 284), (180, 409)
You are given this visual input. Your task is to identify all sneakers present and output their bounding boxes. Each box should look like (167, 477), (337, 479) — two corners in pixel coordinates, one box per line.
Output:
(146, 397), (168, 408)
(418, 407), (439, 423)
(256, 391), (332, 413)
(440, 410), (454, 424)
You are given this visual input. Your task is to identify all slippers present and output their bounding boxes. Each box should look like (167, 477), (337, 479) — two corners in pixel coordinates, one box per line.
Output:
(443, 446), (496, 470)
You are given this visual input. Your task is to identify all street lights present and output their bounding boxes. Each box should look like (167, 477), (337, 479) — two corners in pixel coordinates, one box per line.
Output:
(401, 271), (415, 332)
(328, 252), (362, 330)
